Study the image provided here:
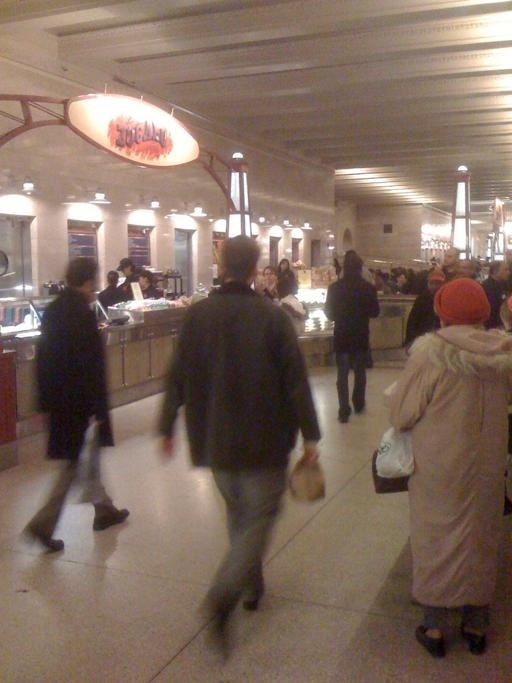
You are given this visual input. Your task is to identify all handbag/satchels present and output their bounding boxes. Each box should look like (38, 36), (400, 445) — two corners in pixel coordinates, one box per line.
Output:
(372, 425), (414, 493)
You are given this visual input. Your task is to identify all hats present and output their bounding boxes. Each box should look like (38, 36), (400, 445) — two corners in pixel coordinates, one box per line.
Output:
(433, 277), (491, 325)
(426, 269), (444, 281)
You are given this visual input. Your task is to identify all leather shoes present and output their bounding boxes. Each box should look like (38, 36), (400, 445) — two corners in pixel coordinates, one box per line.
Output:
(93, 508), (129, 530)
(460, 625), (486, 655)
(25, 526), (63, 551)
(415, 625), (445, 657)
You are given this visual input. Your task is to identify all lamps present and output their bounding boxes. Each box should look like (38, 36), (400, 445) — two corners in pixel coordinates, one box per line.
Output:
(9, 172), (315, 232)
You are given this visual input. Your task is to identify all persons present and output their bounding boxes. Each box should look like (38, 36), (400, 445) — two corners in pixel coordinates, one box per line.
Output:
(326, 249), (380, 423)
(368, 247), (510, 355)
(19, 256), (129, 553)
(256, 258), (299, 300)
(156, 233), (324, 658)
(383, 275), (510, 657)
(102, 257), (157, 314)
